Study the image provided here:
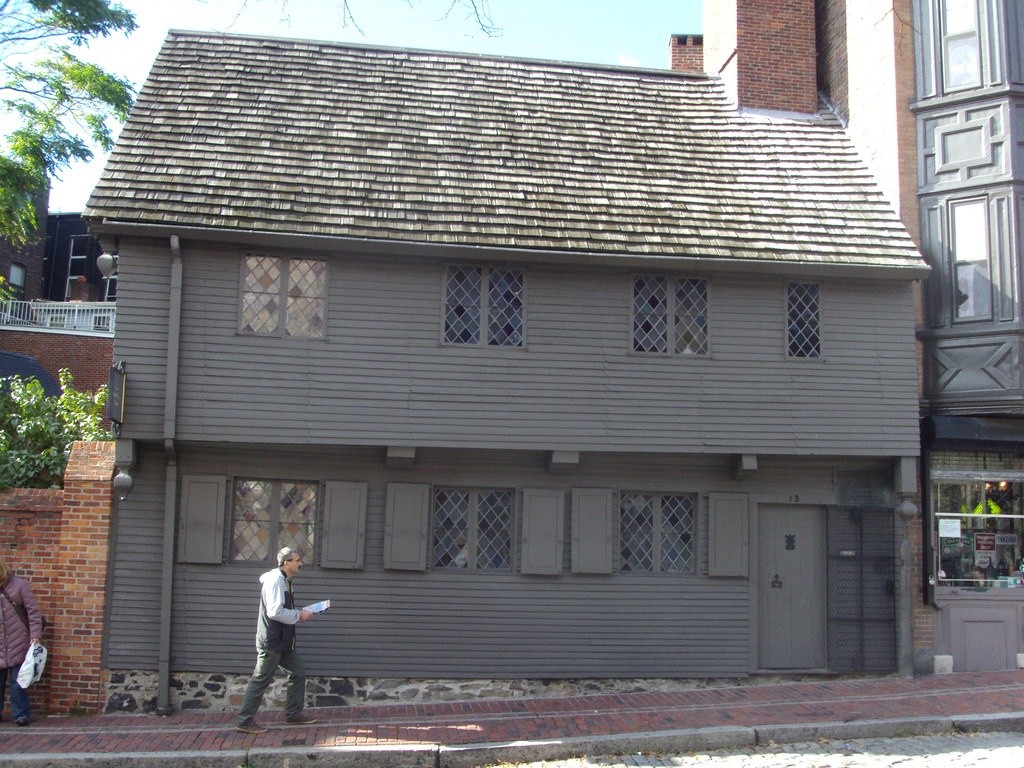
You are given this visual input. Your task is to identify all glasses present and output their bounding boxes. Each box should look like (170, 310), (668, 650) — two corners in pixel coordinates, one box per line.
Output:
(286, 558), (302, 563)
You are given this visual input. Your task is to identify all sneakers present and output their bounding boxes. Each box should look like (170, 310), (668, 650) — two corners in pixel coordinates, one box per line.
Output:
(284, 716), (318, 725)
(233, 720), (268, 733)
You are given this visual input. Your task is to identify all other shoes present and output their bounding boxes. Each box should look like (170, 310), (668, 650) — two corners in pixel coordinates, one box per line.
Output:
(16, 720), (27, 727)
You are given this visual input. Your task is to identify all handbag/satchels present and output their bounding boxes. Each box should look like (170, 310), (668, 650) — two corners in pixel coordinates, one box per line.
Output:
(0, 583), (47, 635)
(16, 642), (48, 689)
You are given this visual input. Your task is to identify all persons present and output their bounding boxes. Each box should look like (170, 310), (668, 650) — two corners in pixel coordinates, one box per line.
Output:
(234, 547), (316, 733)
(0, 562), (42, 725)
